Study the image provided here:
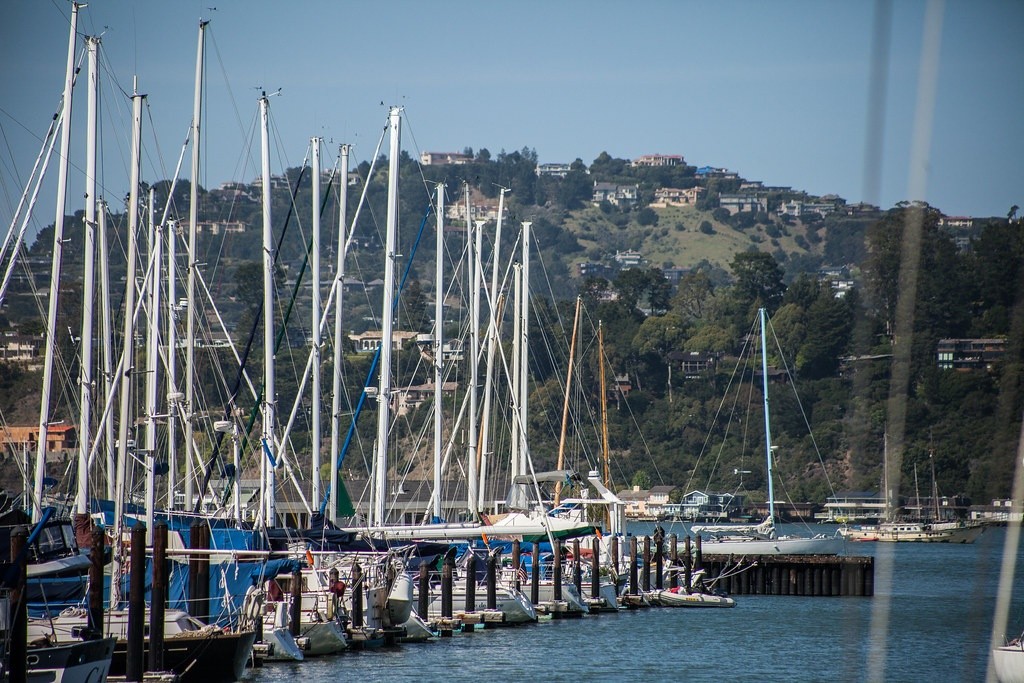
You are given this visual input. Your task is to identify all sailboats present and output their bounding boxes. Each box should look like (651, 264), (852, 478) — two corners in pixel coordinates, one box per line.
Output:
(668, 308), (852, 556)
(836, 429), (989, 544)
(1, 1), (667, 682)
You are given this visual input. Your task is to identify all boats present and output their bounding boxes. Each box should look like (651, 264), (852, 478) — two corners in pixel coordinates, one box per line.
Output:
(660, 590), (738, 608)
(690, 516), (770, 534)
